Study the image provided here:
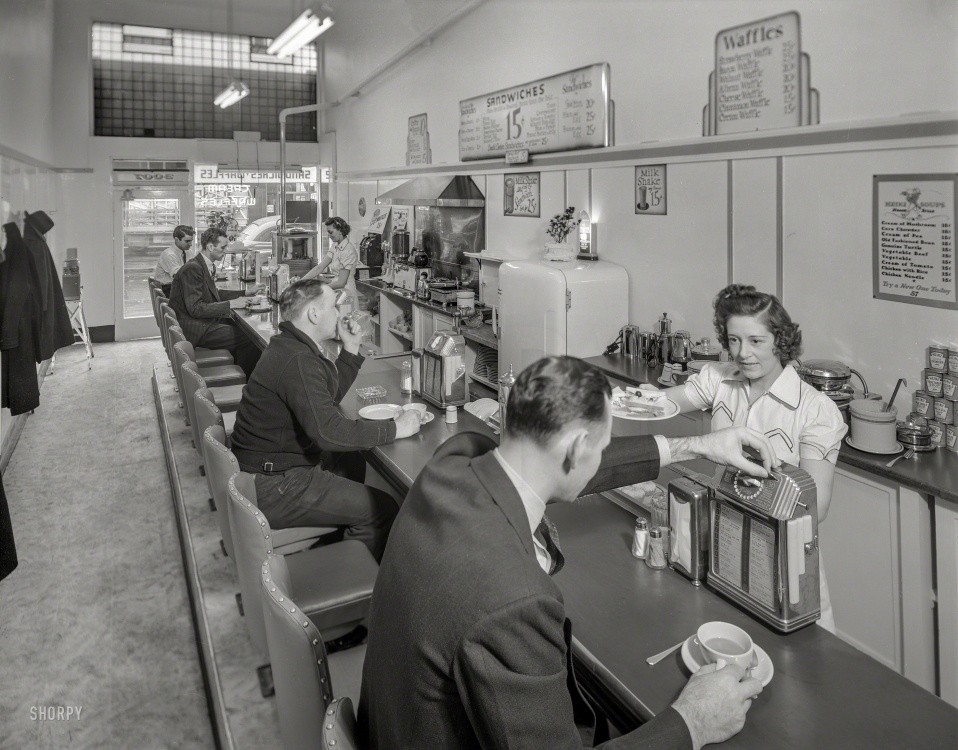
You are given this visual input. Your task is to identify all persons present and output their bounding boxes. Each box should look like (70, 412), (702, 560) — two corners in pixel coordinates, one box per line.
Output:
(153, 225), (195, 299)
(637, 284), (849, 636)
(299, 216), (361, 311)
(355, 354), (781, 750)
(168, 229), (262, 384)
(229, 279), (422, 568)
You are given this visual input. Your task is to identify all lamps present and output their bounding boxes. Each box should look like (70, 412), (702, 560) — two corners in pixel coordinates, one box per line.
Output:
(265, 0), (338, 62)
(212, 0), (253, 110)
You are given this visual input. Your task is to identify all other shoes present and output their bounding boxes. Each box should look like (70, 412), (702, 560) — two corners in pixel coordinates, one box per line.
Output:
(326, 624), (368, 654)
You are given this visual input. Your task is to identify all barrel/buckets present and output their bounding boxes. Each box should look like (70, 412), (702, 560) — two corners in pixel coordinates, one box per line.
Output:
(848, 399), (899, 452)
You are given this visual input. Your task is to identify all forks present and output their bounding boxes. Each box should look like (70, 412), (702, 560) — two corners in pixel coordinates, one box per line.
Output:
(886, 448), (915, 467)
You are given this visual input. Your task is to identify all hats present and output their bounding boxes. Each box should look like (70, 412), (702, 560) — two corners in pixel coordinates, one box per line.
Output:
(24, 209), (54, 243)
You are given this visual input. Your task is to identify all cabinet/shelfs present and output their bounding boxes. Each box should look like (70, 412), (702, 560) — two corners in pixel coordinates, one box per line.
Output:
(355, 277), (958, 711)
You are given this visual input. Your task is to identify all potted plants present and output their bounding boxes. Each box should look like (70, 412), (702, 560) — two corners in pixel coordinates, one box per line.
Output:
(543, 205), (583, 261)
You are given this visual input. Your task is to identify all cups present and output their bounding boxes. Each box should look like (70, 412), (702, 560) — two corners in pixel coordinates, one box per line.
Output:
(216, 272), (225, 277)
(697, 621), (754, 671)
(403, 403), (427, 420)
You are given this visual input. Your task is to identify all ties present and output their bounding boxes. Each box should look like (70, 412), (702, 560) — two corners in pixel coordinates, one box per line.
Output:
(183, 251), (186, 264)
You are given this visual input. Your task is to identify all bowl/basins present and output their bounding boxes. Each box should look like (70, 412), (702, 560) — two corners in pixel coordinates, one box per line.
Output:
(896, 421), (935, 446)
(687, 360), (714, 376)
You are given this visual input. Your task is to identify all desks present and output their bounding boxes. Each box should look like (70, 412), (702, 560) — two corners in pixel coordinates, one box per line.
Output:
(49, 288), (95, 374)
(228, 298), (958, 750)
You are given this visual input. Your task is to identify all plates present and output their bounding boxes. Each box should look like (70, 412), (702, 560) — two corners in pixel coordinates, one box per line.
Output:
(612, 396), (680, 421)
(358, 403), (402, 420)
(898, 440), (937, 451)
(393, 411), (435, 424)
(466, 398), (499, 421)
(680, 634), (775, 687)
(657, 376), (675, 387)
(247, 304), (273, 312)
(217, 277), (228, 279)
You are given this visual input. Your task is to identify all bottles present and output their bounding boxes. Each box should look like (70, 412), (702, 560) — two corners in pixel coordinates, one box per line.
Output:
(644, 528), (667, 569)
(446, 405), (457, 424)
(631, 517), (649, 559)
(661, 363), (672, 382)
(671, 363), (682, 384)
(691, 337), (720, 361)
(400, 361), (412, 393)
(620, 313), (675, 365)
(669, 330), (693, 371)
(414, 249), (429, 267)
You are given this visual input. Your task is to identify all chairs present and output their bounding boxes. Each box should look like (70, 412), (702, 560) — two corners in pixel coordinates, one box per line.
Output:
(145, 274), (382, 750)
